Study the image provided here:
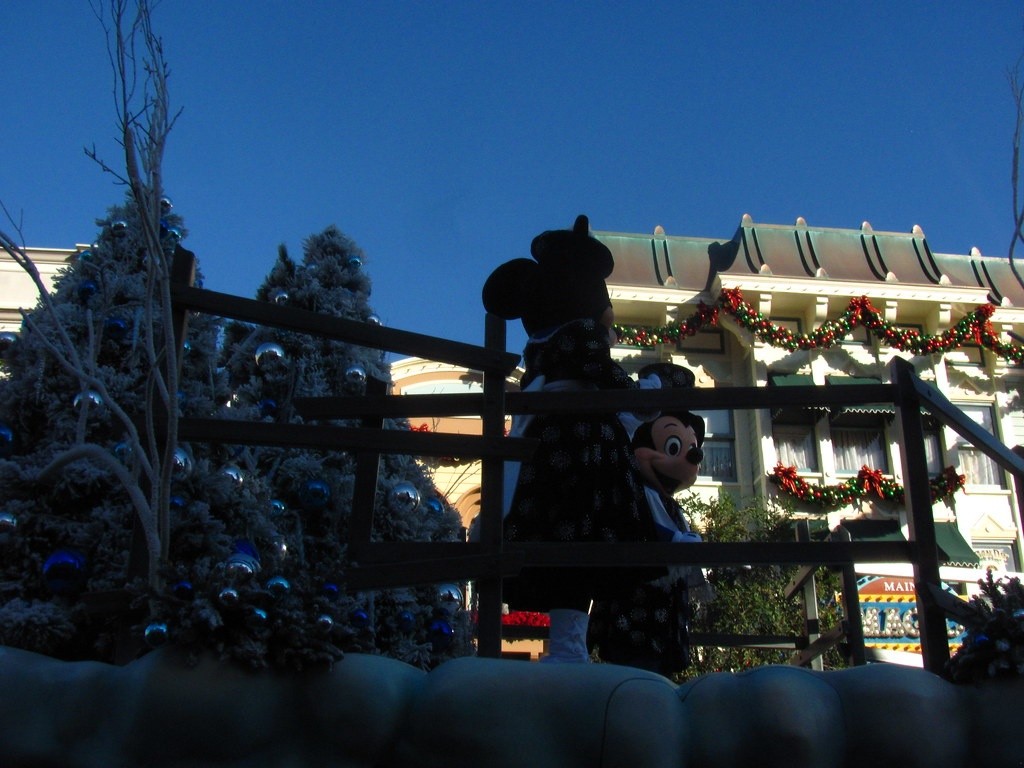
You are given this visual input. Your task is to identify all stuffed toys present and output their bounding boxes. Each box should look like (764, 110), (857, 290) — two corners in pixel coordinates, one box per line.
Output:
(481, 214), (703, 675)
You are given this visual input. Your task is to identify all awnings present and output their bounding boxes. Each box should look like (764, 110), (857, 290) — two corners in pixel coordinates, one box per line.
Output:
(785, 517), (980, 568)
(769, 373), (950, 420)
